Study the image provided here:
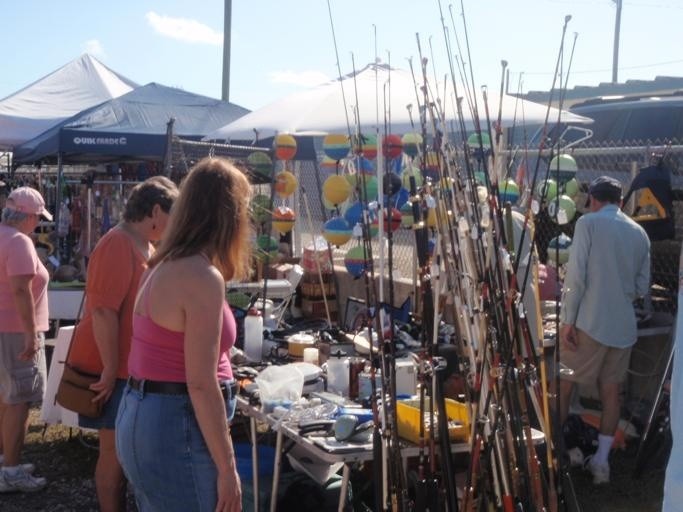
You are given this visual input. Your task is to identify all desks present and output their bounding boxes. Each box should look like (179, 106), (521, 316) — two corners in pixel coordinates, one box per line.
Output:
(236, 390), (546, 512)
(45, 280), (293, 348)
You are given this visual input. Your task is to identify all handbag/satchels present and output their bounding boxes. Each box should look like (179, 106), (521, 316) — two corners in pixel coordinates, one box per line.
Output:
(55, 364), (102, 417)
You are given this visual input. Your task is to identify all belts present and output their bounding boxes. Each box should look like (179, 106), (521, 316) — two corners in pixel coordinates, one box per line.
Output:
(129, 380), (238, 400)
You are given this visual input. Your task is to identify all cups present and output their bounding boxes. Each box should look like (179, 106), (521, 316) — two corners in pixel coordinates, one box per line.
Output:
(287, 331), (385, 406)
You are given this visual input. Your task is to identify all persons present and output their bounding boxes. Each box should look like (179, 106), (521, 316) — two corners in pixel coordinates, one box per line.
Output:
(0, 185), (53, 493)
(549, 176), (652, 484)
(58, 201), (74, 260)
(114, 157), (254, 512)
(69, 175), (179, 512)
(454, 179), (543, 362)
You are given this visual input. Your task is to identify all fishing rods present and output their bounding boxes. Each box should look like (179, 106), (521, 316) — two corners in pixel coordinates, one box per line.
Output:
(328, 0), (575, 511)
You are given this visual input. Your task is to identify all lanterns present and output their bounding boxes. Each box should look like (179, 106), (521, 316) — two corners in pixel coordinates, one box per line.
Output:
(244, 131), (298, 266)
(498, 176), (522, 205)
(468, 130), (493, 159)
(319, 127), (454, 281)
(472, 173), (490, 185)
(540, 153), (578, 264)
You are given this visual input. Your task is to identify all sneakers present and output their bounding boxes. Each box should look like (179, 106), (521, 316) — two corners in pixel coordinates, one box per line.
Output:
(587, 457), (611, 485)
(553, 447), (584, 472)
(1, 461), (47, 493)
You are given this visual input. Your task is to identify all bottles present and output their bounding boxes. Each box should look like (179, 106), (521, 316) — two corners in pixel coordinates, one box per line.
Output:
(244, 307), (262, 363)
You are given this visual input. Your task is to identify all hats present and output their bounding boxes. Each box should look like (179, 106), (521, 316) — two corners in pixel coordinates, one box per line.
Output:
(7, 187), (53, 222)
(584, 176), (621, 208)
